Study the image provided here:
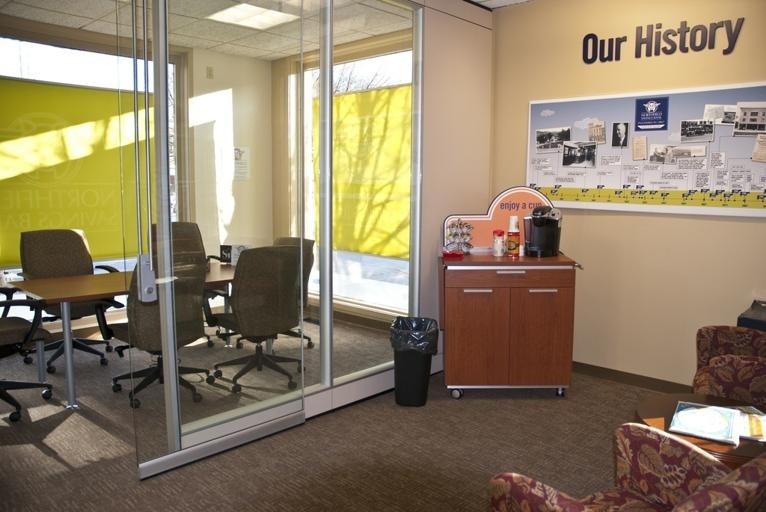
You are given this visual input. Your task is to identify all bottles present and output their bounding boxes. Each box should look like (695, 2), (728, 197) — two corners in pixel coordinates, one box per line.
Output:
(492, 215), (520, 259)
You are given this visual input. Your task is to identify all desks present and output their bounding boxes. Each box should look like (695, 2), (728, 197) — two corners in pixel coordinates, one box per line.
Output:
(8, 263), (276, 411)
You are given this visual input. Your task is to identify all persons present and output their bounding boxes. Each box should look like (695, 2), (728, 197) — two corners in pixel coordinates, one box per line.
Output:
(615, 123), (626, 146)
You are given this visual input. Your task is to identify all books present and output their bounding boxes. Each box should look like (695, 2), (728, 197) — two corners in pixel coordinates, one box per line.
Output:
(668, 399), (766, 447)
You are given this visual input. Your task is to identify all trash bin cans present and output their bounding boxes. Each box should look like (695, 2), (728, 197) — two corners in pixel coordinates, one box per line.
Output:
(389, 316), (439, 407)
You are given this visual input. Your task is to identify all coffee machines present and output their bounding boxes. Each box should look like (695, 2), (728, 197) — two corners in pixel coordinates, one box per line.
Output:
(524, 205), (562, 257)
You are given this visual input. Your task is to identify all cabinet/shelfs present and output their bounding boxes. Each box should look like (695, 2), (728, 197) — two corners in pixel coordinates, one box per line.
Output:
(437, 251), (581, 400)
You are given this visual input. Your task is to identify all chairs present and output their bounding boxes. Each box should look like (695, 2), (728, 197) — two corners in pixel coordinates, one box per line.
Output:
(485, 422), (766, 512)
(216, 237), (314, 358)
(17, 229), (124, 372)
(213, 244), (312, 393)
(692, 325), (766, 416)
(109, 221), (213, 409)
(0, 285), (54, 422)
